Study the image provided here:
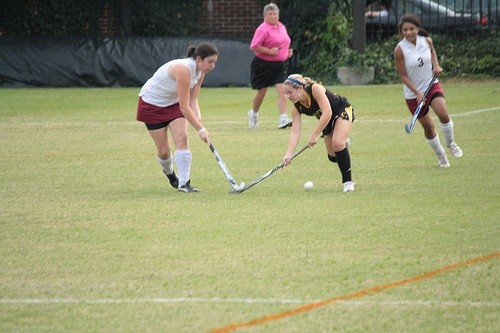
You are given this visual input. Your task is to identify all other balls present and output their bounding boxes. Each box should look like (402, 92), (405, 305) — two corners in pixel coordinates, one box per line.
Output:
(303, 181), (314, 191)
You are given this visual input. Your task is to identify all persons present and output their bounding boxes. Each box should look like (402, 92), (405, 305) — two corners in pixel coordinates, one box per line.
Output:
(364, 0), (389, 17)
(393, 14), (462, 168)
(137, 43), (219, 191)
(247, 3), (293, 129)
(282, 73), (354, 193)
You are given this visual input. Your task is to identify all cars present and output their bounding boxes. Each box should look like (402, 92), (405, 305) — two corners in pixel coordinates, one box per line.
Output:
(347, 0), (489, 45)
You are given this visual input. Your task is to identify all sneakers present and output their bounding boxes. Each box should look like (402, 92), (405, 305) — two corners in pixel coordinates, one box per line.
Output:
(436, 149), (450, 168)
(344, 137), (351, 151)
(278, 118), (293, 130)
(447, 140), (463, 159)
(162, 168), (180, 188)
(248, 111), (260, 128)
(178, 180), (200, 193)
(343, 180), (355, 193)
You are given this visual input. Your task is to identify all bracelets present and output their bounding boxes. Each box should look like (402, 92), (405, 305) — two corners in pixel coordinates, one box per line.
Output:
(198, 128), (206, 134)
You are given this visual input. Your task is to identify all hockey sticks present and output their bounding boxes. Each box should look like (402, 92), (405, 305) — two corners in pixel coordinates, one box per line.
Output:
(205, 138), (246, 191)
(227, 142), (322, 195)
(405, 72), (437, 134)
